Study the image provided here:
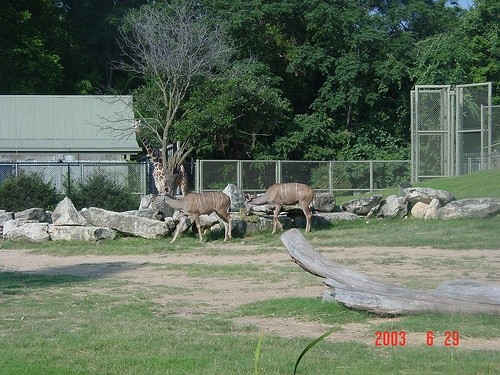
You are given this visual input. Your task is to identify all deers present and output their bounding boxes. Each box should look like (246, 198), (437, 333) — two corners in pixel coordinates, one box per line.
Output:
(152, 186), (235, 245)
(244, 182), (317, 235)
